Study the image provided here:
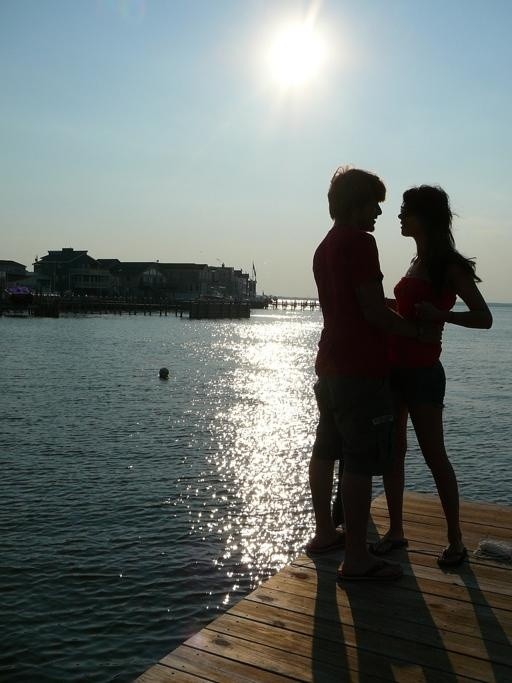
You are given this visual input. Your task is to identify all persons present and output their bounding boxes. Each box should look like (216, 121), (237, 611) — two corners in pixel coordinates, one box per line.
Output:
(304, 160), (443, 589)
(367, 182), (496, 573)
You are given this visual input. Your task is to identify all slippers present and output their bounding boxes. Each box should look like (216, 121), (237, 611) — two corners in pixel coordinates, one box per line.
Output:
(368, 533), (409, 556)
(338, 558), (403, 581)
(437, 547), (467, 571)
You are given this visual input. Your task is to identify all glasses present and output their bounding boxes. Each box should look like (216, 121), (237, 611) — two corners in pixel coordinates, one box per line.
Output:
(306, 529), (345, 553)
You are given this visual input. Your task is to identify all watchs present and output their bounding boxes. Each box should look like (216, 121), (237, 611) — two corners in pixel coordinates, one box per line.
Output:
(414, 322), (424, 340)
